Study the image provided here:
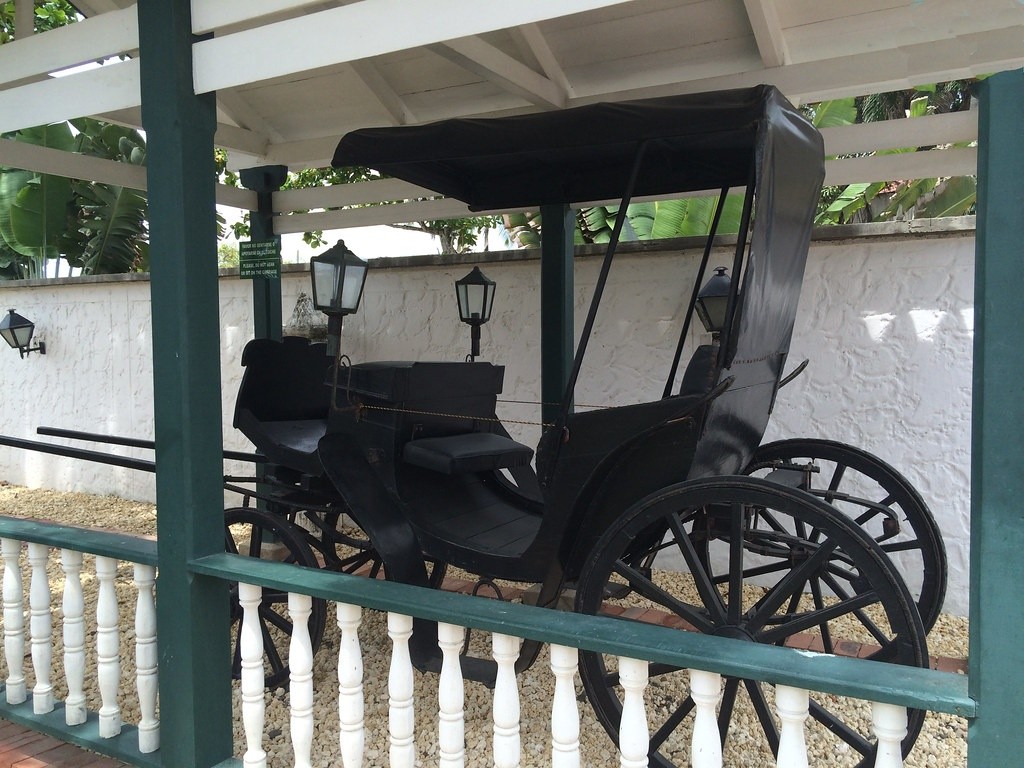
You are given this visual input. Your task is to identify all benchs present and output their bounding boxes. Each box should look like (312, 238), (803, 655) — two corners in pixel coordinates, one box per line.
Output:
(536, 344), (720, 500)
(401, 430), (534, 475)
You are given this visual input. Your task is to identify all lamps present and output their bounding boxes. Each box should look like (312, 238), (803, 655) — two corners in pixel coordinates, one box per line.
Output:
(695, 266), (741, 332)
(456, 266), (496, 361)
(310, 239), (364, 417)
(0, 309), (46, 358)
